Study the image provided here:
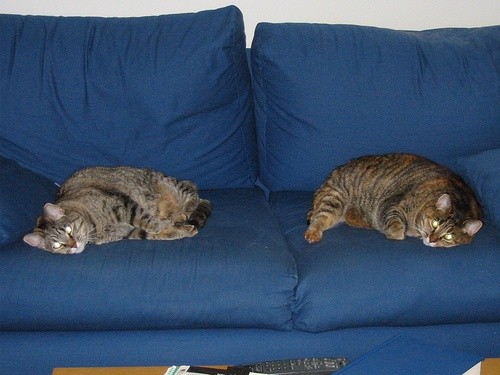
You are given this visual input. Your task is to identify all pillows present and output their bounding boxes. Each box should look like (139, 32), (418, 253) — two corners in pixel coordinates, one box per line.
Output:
(0, 153), (62, 251)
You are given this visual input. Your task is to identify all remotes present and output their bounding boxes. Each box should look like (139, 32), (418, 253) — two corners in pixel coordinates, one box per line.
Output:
(234, 357), (349, 375)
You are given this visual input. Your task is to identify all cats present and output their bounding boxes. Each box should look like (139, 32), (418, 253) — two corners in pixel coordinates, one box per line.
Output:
(22, 165), (213, 256)
(304, 153), (484, 248)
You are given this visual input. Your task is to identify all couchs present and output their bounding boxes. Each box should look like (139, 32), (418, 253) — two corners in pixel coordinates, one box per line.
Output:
(0, 4), (499, 374)
